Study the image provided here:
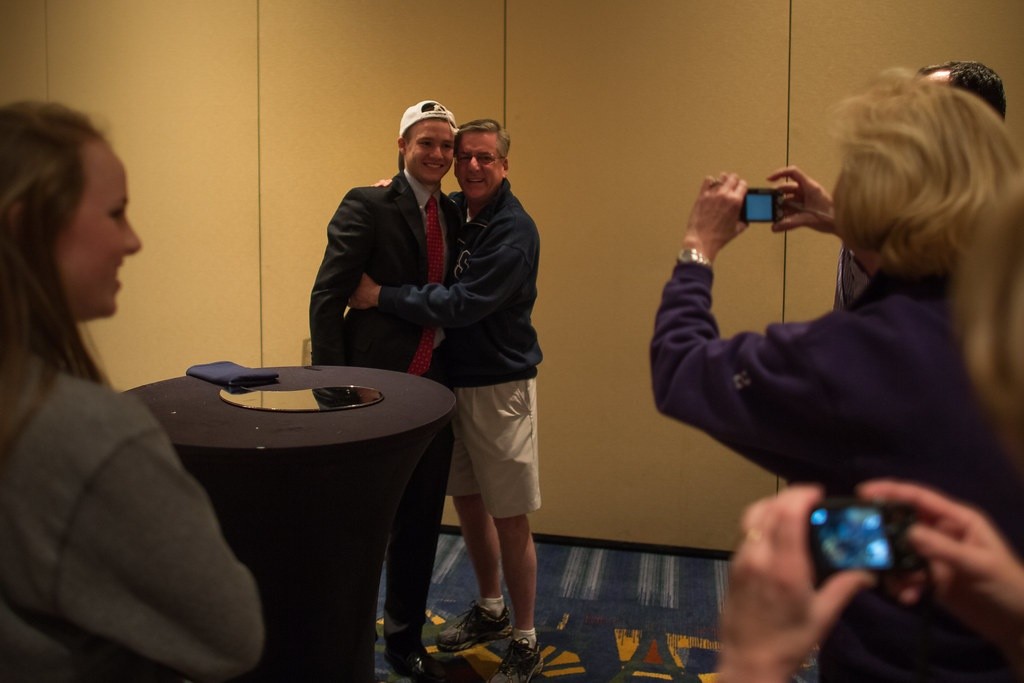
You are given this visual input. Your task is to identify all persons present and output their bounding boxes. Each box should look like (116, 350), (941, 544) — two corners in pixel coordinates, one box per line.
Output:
(2, 101), (264, 681)
(649, 61), (1024, 682)
(309, 100), (543, 683)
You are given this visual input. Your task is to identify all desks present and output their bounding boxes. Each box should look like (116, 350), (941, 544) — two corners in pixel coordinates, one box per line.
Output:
(117, 365), (458, 683)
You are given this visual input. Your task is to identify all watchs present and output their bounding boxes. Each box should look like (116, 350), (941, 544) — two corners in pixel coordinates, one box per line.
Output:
(676, 248), (714, 266)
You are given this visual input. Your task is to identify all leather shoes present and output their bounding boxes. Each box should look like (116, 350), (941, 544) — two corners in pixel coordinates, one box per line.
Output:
(384, 648), (446, 682)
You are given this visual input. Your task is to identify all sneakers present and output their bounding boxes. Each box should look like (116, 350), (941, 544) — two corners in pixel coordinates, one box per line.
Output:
(487, 637), (543, 683)
(437, 600), (513, 652)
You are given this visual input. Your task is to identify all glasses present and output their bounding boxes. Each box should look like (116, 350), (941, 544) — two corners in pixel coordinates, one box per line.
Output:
(453, 151), (505, 165)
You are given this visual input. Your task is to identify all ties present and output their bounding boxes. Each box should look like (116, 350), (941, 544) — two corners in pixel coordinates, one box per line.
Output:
(408, 196), (444, 376)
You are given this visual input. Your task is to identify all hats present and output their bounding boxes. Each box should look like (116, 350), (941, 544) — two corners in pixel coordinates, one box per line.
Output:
(397, 100), (456, 171)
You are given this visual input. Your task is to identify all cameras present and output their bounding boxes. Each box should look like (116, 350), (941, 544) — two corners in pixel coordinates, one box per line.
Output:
(805, 496), (926, 587)
(737, 188), (782, 222)
